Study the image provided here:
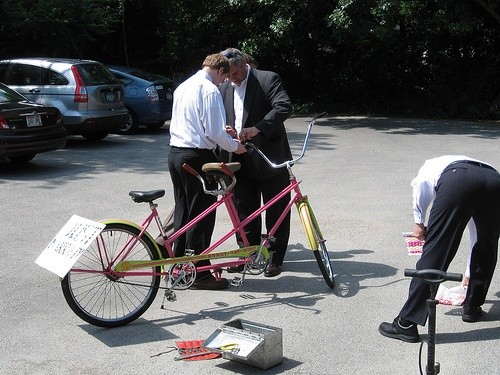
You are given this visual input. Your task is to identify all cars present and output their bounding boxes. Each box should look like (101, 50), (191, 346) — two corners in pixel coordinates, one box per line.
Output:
(0, 80), (68, 166)
(106, 64), (180, 135)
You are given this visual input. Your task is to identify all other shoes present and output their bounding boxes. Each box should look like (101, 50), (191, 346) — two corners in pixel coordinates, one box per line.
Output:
(174, 275), (187, 284)
(189, 274), (228, 289)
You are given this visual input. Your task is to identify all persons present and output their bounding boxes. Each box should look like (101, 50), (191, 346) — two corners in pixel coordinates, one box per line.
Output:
(379, 155), (500, 343)
(168, 53), (247, 290)
(219, 48), (295, 278)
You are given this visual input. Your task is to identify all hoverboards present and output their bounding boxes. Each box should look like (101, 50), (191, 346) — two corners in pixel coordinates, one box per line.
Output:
(402, 265), (464, 375)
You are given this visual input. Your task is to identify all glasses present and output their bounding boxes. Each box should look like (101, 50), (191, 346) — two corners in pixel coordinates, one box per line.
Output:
(221, 74), (227, 83)
(225, 53), (233, 59)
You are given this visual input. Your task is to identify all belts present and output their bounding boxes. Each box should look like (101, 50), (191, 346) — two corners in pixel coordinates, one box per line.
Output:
(448, 160), (496, 171)
(170, 146), (211, 152)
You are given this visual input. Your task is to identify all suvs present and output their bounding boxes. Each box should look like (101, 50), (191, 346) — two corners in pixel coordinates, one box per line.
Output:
(0, 55), (130, 142)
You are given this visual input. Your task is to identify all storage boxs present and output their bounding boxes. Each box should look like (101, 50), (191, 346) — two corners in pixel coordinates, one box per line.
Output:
(200, 317), (283, 370)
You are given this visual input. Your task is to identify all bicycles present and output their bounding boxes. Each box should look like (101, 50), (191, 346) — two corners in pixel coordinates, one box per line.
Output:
(58, 108), (337, 328)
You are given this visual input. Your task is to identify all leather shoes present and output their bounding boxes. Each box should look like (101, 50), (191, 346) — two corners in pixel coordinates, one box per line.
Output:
(227, 258), (253, 272)
(264, 262), (281, 277)
(462, 303), (482, 322)
(378, 316), (419, 342)
